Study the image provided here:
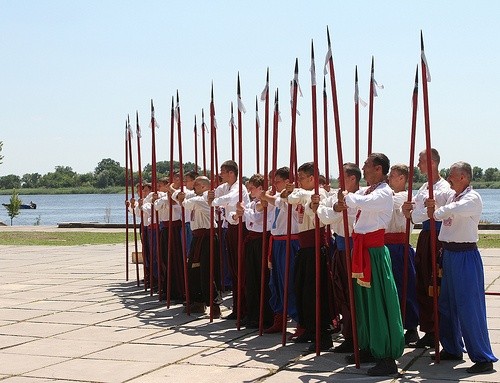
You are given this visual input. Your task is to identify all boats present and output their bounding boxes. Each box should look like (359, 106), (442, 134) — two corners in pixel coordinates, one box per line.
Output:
(2, 200), (36, 209)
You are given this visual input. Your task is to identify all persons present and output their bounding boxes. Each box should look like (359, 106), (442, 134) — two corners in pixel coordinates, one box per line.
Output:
(421, 161), (499, 373)
(209, 169), (344, 335)
(316, 163), (362, 353)
(383, 164), (420, 345)
(171, 176), (223, 319)
(280, 162), (334, 353)
(399, 148), (457, 348)
(251, 168), (306, 341)
(202, 160), (251, 320)
(332, 153), (405, 376)
(125, 171), (201, 305)
(227, 174), (274, 328)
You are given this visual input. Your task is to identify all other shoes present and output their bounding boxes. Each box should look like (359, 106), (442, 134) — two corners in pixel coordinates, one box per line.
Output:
(294, 330), (315, 343)
(308, 333), (334, 352)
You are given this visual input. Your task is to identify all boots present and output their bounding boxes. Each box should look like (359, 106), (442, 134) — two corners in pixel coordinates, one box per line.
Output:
(289, 321), (306, 341)
(263, 312), (283, 334)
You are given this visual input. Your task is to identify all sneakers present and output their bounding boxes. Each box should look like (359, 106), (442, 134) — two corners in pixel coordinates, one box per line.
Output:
(467, 361), (494, 374)
(409, 330), (435, 348)
(183, 302), (204, 313)
(404, 328), (420, 343)
(334, 338), (355, 352)
(367, 359), (397, 376)
(346, 347), (371, 364)
(213, 305), (220, 319)
(431, 349), (464, 360)
